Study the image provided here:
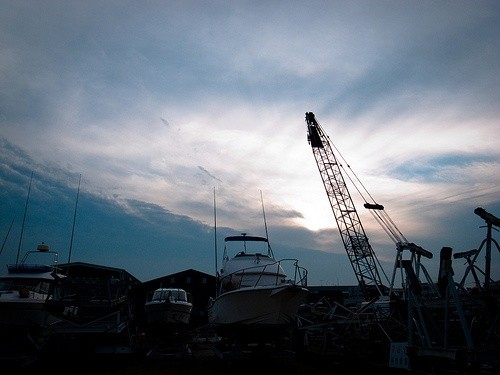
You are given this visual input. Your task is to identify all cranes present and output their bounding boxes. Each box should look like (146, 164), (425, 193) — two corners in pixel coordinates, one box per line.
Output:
(304, 111), (411, 303)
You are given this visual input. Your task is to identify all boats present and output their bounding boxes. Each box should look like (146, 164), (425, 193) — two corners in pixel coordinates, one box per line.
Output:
(144, 287), (192, 326)
(0, 241), (79, 326)
(208, 232), (308, 328)
